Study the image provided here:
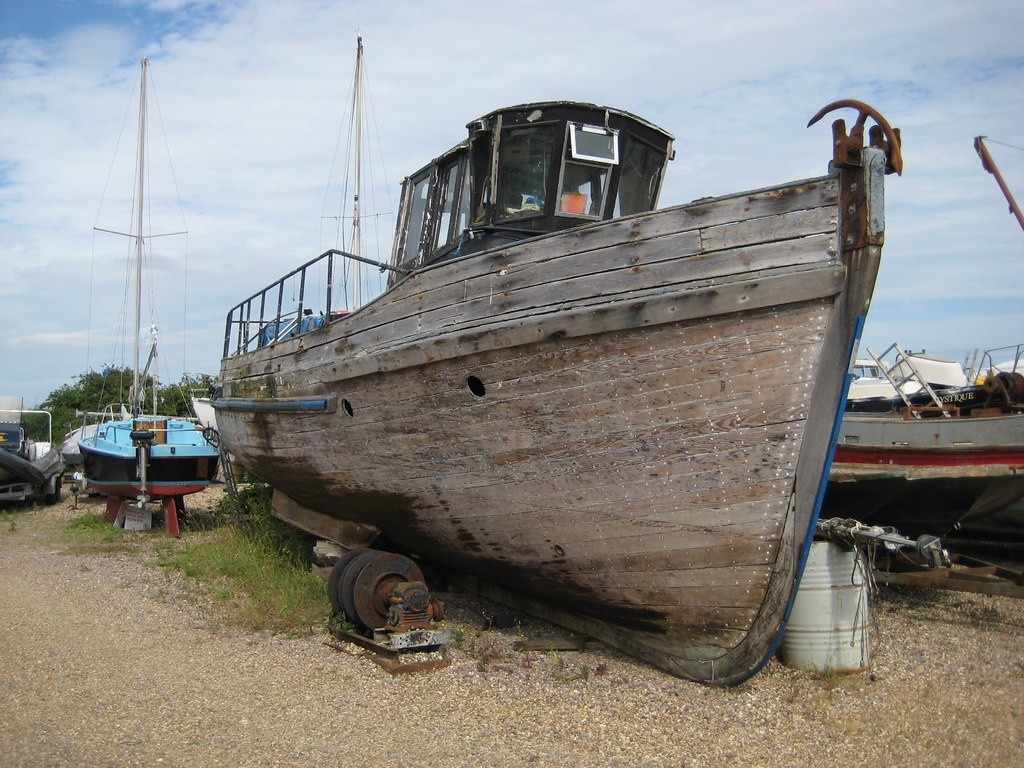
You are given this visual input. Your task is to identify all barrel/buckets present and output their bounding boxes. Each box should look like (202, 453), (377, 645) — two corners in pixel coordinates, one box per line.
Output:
(783, 537), (872, 680)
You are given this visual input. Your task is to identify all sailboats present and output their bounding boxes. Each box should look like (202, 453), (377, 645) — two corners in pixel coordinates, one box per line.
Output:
(73, 60), (221, 499)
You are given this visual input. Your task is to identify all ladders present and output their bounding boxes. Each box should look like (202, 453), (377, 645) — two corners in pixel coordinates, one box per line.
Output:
(866, 343), (950, 419)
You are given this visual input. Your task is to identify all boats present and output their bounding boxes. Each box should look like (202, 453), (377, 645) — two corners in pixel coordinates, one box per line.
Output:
(62, 424), (101, 478)
(0, 407), (66, 508)
(217, 98), (904, 689)
(810, 372), (1023, 554)
(182, 372), (219, 435)
